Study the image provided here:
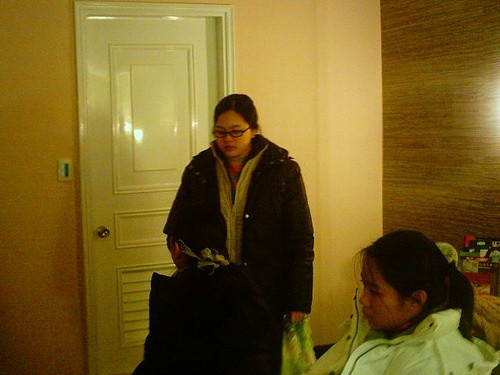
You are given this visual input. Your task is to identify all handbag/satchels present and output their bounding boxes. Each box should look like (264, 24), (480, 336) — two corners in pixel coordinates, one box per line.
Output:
(141, 267), (275, 375)
(280, 313), (318, 375)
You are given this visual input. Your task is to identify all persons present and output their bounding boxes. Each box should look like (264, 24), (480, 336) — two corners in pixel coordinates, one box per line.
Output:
(164, 95), (317, 324)
(303, 229), (500, 375)
(130, 202), (282, 375)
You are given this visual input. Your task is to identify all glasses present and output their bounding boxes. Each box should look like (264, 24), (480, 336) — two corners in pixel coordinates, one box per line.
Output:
(212, 123), (253, 138)
